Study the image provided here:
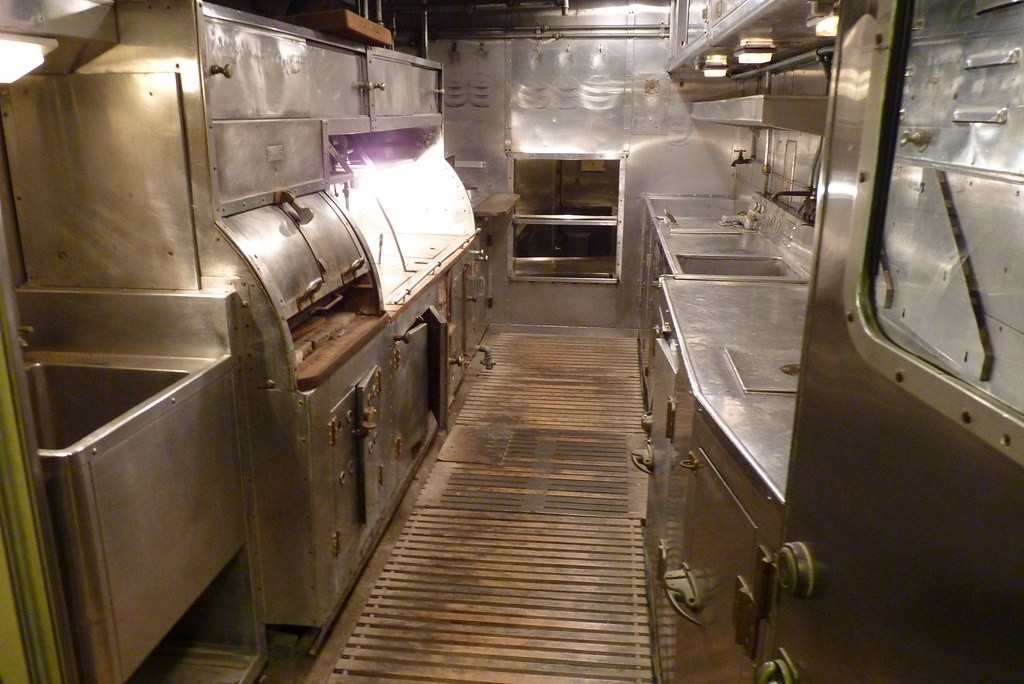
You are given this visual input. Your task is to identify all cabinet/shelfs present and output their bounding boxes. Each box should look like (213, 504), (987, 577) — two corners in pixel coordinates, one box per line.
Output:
(643, 277), (683, 576)
(661, 394), (782, 684)
(447, 246), (478, 391)
(309, 314), (396, 630)
(398, 273), (452, 490)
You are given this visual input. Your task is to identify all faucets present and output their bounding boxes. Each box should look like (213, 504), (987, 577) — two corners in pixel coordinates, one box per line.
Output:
(731, 148), (754, 169)
(770, 188), (815, 202)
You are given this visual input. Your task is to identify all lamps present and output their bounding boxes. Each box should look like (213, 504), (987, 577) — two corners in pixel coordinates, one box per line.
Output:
(733, 35), (779, 66)
(699, 63), (732, 79)
(813, 2), (841, 39)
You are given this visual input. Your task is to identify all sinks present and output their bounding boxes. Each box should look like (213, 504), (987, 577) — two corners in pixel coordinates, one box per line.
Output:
(650, 196), (754, 222)
(11, 350), (218, 458)
(675, 253), (808, 280)
